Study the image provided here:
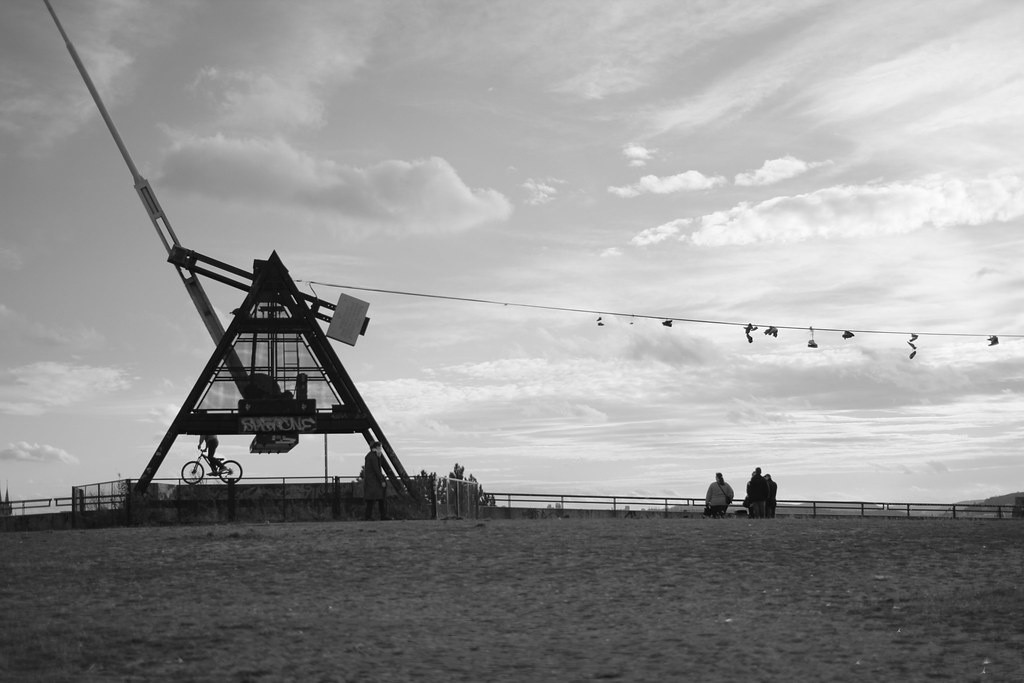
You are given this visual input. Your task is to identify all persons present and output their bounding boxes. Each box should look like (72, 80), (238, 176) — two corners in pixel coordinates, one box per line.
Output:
(197, 410), (226, 475)
(365, 442), (392, 520)
(706, 473), (734, 518)
(747, 467), (777, 519)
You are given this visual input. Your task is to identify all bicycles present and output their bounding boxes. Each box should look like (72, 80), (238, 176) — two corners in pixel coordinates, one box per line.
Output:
(180, 447), (243, 484)
